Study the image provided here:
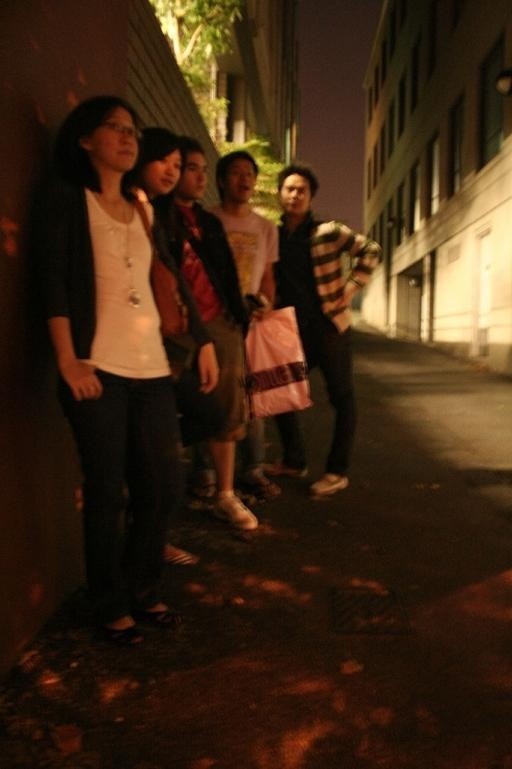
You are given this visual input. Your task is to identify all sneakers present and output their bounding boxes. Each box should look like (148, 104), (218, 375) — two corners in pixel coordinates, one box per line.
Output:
(213, 494), (259, 530)
(263, 462), (308, 478)
(308, 472), (349, 494)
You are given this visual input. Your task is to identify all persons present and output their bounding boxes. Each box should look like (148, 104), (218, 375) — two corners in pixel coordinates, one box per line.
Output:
(157, 135), (259, 531)
(257, 163), (382, 496)
(190, 150), (283, 511)
(37, 94), (221, 645)
(118, 125), (202, 568)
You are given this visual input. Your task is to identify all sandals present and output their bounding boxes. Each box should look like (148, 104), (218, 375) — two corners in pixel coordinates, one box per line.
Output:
(189, 481), (218, 511)
(89, 605), (145, 645)
(144, 597), (183, 628)
(161, 543), (200, 567)
(241, 469), (282, 498)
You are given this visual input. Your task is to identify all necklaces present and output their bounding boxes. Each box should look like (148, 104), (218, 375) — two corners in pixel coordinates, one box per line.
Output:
(95, 191), (143, 308)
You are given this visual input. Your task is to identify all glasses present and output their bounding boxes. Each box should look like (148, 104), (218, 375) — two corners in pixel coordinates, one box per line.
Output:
(105, 119), (142, 139)
